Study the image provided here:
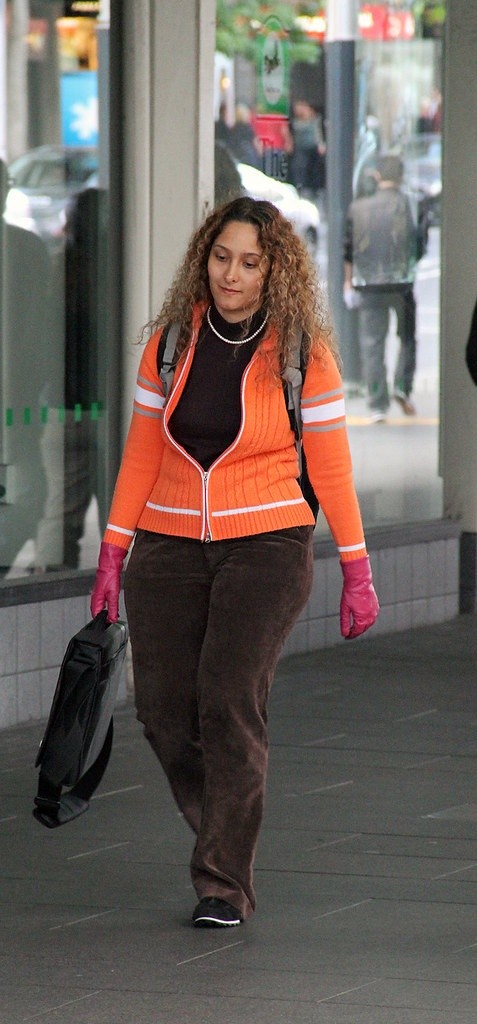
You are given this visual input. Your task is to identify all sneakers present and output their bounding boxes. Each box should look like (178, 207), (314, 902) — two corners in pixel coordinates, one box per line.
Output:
(372, 411), (385, 424)
(192, 897), (241, 926)
(393, 390), (415, 415)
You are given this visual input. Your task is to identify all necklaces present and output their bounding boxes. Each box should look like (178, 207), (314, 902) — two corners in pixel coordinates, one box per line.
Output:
(206, 305), (270, 345)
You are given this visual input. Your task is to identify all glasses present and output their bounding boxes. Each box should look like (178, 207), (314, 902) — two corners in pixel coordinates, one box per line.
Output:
(6, 178), (17, 189)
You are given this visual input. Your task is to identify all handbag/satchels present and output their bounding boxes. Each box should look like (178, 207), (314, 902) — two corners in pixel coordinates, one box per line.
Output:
(32, 610), (129, 829)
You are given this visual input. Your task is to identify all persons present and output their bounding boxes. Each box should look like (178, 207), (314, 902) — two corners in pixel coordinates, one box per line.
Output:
(342, 156), (430, 423)
(230, 103), (258, 169)
(37, 188), (106, 578)
(90, 196), (379, 927)
(214, 102), (229, 140)
(290, 98), (328, 198)
(423, 82), (444, 130)
(0, 157), (52, 577)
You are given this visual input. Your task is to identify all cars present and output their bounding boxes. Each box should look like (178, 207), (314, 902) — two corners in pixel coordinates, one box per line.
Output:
(360, 131), (442, 233)
(2, 143), (321, 273)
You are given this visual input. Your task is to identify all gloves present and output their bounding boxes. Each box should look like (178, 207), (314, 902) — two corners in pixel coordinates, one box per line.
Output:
(338, 555), (380, 640)
(90, 542), (129, 623)
